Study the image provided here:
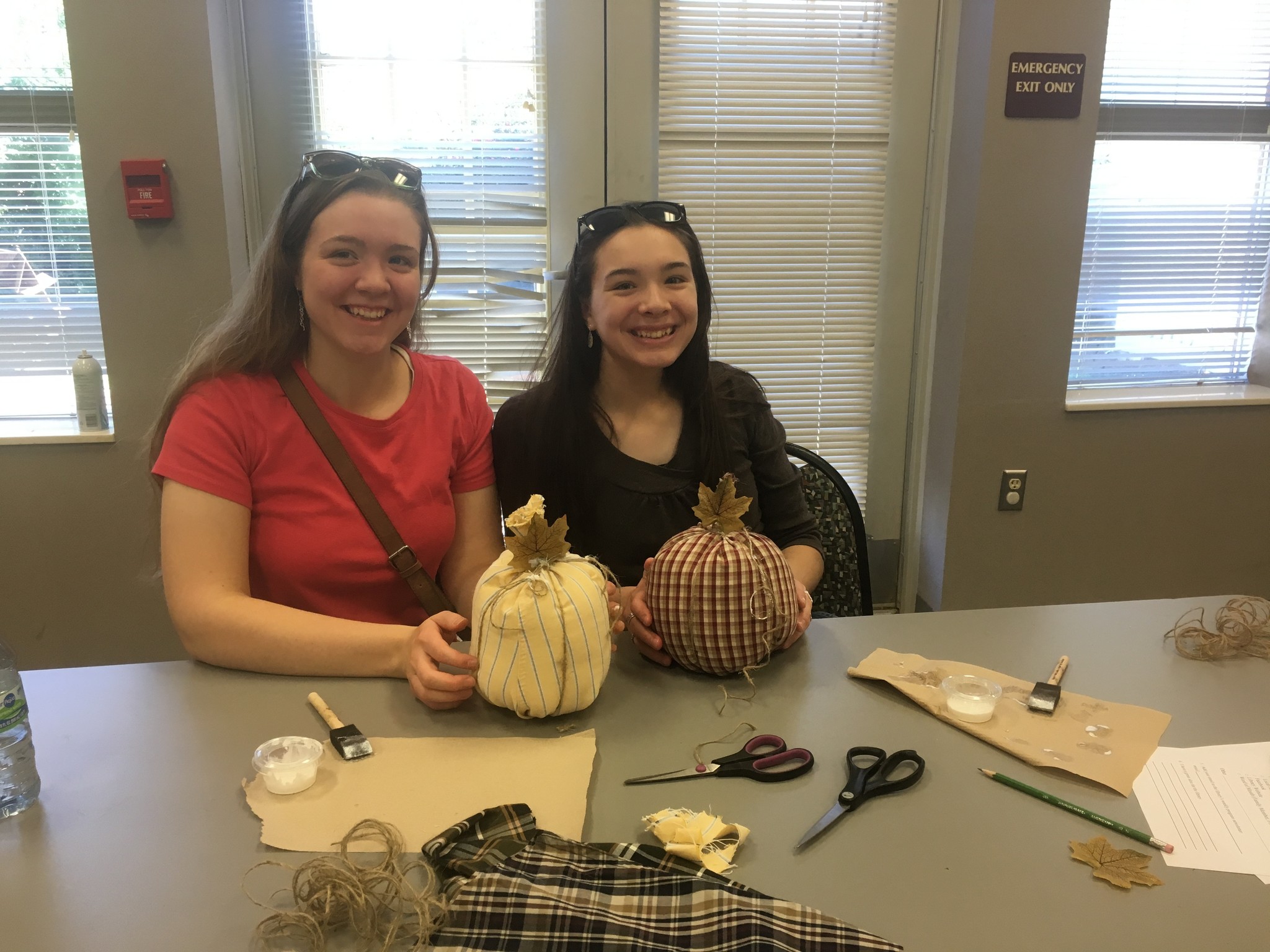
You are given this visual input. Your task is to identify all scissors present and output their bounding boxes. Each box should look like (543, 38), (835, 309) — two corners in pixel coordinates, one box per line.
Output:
(624, 735), (814, 785)
(797, 747), (925, 848)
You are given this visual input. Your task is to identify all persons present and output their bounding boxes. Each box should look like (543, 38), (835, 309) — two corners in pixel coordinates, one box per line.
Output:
(141, 150), (627, 712)
(491, 200), (829, 667)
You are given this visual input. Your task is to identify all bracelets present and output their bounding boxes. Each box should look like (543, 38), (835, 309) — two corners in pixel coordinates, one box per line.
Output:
(804, 590), (813, 622)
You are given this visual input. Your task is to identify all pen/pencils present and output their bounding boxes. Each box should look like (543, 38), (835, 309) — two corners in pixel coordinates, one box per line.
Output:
(977, 768), (1174, 853)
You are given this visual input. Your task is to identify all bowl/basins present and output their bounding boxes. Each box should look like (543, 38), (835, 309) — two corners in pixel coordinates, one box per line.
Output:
(942, 674), (1002, 723)
(251, 736), (323, 795)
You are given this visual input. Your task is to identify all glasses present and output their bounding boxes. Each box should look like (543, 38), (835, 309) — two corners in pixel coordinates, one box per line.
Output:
(577, 202), (687, 252)
(301, 151), (423, 191)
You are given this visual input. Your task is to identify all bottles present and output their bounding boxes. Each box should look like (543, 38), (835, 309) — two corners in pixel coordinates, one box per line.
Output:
(73, 349), (108, 433)
(0, 638), (41, 819)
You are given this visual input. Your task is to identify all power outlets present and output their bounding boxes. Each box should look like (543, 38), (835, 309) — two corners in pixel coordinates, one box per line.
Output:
(997, 467), (1028, 514)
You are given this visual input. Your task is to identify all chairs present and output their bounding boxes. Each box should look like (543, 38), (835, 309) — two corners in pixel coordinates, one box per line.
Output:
(784, 441), (875, 615)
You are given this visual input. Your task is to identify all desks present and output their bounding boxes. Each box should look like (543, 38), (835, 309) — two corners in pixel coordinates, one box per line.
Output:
(0, 596), (1270, 952)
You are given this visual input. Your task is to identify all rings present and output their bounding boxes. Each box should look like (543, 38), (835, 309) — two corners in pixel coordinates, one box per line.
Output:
(631, 634), (636, 644)
(627, 612), (635, 625)
